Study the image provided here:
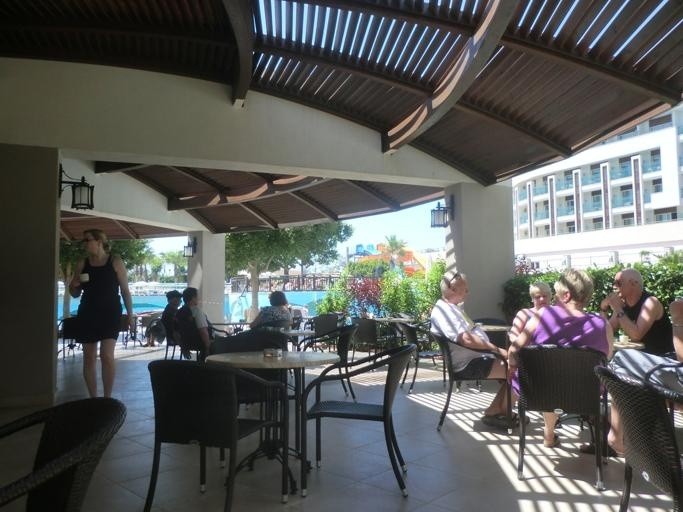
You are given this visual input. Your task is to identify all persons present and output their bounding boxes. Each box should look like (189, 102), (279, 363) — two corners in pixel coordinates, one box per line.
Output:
(142, 317), (167, 349)
(506, 267), (614, 447)
(69, 228), (135, 398)
(249, 291), (294, 329)
(599, 268), (676, 360)
(578, 297), (683, 455)
(509, 281), (553, 342)
(171, 287), (225, 359)
(430, 270), (530, 426)
(162, 290), (183, 330)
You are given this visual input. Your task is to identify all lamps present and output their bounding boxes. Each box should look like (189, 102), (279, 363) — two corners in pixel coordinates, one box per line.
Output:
(431, 194), (454, 227)
(184, 237), (196, 257)
(58, 162), (95, 210)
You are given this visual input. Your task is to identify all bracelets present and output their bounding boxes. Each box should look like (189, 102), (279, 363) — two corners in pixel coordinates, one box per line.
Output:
(600, 307), (608, 312)
(672, 323), (683, 327)
(495, 347), (499, 353)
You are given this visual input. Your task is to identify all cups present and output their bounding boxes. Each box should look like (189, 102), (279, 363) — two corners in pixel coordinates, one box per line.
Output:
(619, 335), (631, 344)
(79, 273), (89, 283)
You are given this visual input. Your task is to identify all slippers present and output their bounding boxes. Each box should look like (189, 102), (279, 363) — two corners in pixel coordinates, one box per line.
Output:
(484, 412), (527, 428)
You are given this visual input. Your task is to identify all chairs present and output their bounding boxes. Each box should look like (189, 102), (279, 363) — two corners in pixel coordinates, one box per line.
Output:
(0, 396), (126, 512)
(142, 360), (289, 512)
(428, 330), (513, 435)
(518, 344), (610, 491)
(299, 344), (418, 497)
(472, 318), (507, 347)
(593, 365), (683, 512)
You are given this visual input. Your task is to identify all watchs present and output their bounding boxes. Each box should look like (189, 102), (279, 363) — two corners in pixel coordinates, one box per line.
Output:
(616, 312), (624, 318)
